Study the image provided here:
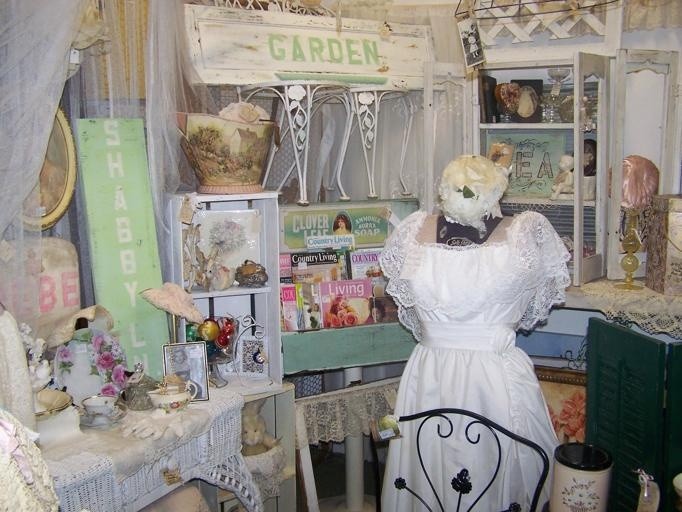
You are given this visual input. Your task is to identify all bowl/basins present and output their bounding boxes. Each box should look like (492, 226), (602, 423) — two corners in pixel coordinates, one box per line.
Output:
(35, 388), (74, 421)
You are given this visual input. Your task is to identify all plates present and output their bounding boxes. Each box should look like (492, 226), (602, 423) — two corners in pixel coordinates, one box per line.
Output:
(74, 401), (127, 424)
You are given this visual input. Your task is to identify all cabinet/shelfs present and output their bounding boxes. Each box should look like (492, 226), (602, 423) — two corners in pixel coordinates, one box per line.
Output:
(469, 56), (604, 279)
(164, 190), (283, 385)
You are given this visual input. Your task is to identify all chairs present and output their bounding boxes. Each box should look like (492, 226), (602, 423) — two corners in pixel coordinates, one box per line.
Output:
(206, 0), (417, 206)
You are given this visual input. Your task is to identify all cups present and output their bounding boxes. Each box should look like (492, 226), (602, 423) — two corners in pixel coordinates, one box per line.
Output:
(545, 95), (561, 123)
(146, 381), (198, 414)
(81, 393), (119, 419)
(581, 176), (595, 201)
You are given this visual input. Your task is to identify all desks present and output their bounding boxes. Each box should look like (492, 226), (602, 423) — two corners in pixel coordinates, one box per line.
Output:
(0, 383), (297, 512)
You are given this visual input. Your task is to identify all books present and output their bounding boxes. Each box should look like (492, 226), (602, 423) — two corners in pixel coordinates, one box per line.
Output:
(273, 246), (398, 329)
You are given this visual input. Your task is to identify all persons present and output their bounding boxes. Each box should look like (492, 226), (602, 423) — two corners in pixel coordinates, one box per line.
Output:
(173, 349), (204, 401)
(378, 152), (572, 512)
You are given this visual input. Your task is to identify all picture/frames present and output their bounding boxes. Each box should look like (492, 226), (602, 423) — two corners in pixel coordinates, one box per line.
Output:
(162, 340), (210, 402)
(2, 93), (78, 229)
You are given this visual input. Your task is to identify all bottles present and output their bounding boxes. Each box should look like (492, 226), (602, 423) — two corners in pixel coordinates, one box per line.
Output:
(125, 361), (157, 410)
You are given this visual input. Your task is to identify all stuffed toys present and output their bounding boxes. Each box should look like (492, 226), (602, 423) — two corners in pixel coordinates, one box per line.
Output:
(241, 398), (279, 456)
